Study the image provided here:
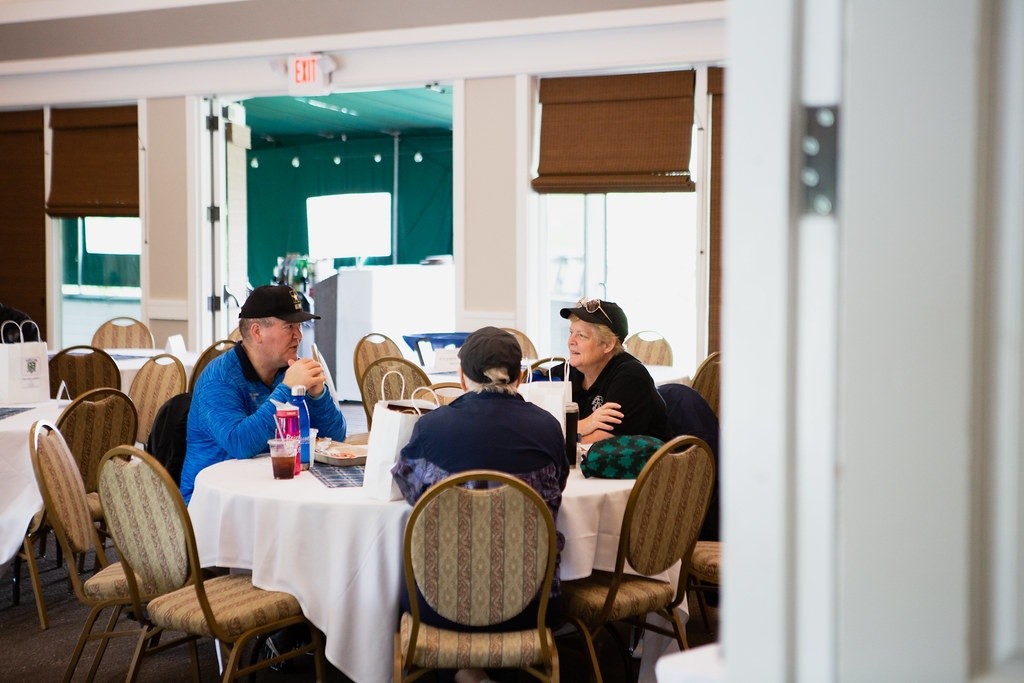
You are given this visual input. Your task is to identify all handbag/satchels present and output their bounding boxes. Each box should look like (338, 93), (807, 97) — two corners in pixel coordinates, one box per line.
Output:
(518, 353), (573, 441)
(363, 370), (439, 501)
(579, 435), (669, 480)
(0, 319), (51, 408)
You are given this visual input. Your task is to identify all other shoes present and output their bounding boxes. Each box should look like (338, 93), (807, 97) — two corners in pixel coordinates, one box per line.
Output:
(258, 627), (316, 671)
(552, 621), (577, 636)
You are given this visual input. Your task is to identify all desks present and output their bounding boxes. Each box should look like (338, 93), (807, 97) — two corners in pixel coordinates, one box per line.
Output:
(0, 396), (76, 609)
(184, 435), (636, 683)
(522, 360), (682, 389)
(98, 350), (201, 394)
(402, 332), (473, 367)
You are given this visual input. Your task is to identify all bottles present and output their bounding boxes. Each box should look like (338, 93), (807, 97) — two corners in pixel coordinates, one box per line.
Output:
(566, 402), (579, 469)
(290, 385), (310, 469)
(271, 399), (301, 474)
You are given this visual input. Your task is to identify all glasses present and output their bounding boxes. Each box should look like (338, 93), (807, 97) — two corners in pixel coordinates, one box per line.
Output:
(577, 296), (613, 325)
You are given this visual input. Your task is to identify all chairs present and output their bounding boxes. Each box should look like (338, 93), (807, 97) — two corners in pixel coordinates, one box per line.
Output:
(687, 539), (719, 651)
(624, 329), (673, 368)
(353, 334), (404, 431)
(502, 327), (540, 368)
(146, 393), (193, 490)
(393, 466), (560, 683)
(549, 434), (716, 683)
(96, 445), (326, 683)
(519, 357), (567, 386)
(187, 339), (239, 393)
(223, 326), (245, 351)
(362, 356), (433, 425)
(30, 419), (201, 683)
(91, 316), (155, 350)
(16, 385), (141, 631)
(412, 381), (464, 406)
(312, 342), (341, 411)
(690, 354), (720, 418)
(94, 352), (186, 575)
(48, 345), (122, 402)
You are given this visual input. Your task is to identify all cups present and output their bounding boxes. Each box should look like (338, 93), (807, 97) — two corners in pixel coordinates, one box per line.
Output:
(267, 438), (301, 479)
(306, 428), (318, 466)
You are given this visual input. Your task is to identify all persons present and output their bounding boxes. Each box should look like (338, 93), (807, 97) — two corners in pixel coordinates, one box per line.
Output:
(547, 299), (683, 637)
(0, 303), (38, 344)
(391, 326), (571, 683)
(178, 285), (348, 671)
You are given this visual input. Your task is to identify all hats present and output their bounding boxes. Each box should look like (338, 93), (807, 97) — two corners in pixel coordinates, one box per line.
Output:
(458, 326), (523, 385)
(240, 285), (321, 324)
(560, 300), (628, 343)
